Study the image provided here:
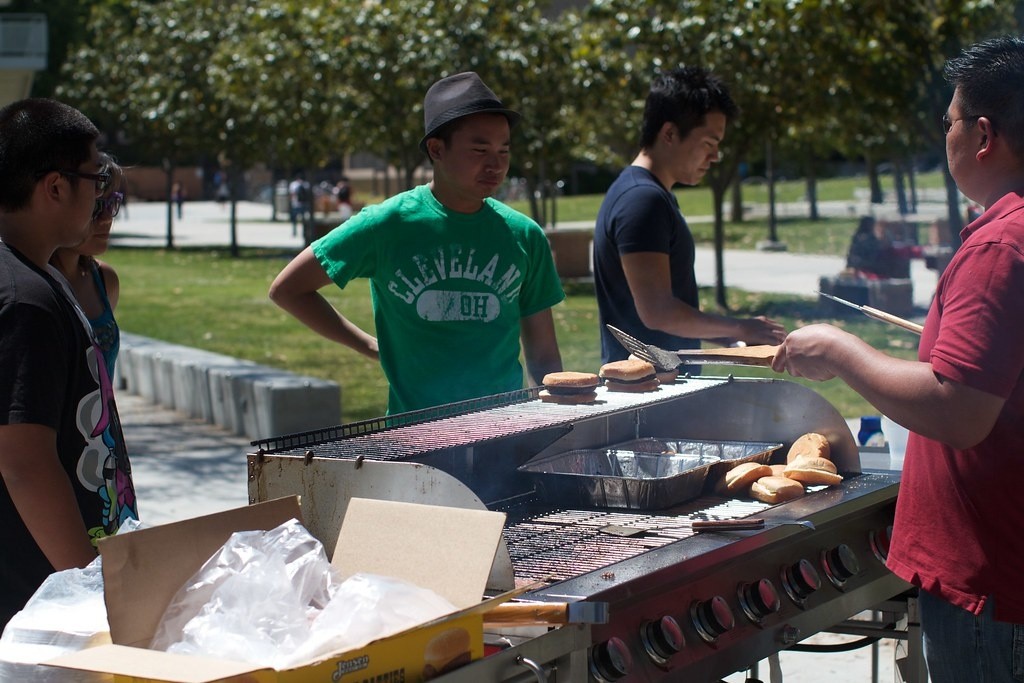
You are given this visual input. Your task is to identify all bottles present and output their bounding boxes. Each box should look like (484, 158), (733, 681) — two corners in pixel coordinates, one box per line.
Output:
(856, 415), (885, 449)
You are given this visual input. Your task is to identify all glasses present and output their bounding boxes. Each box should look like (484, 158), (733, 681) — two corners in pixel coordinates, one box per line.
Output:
(91, 191), (126, 223)
(942, 114), (999, 137)
(40, 169), (111, 194)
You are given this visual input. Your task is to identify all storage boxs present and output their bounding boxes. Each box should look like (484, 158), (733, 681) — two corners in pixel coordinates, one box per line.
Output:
(38, 495), (537, 682)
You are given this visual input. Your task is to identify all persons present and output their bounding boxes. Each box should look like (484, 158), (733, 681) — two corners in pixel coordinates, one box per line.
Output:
(593, 65), (786, 377)
(267, 72), (569, 429)
(286, 174), (352, 237)
(845, 215), (890, 274)
(0, 98), (138, 636)
(171, 178), (186, 219)
(217, 174), (231, 216)
(47, 153), (124, 395)
(770, 34), (1024, 683)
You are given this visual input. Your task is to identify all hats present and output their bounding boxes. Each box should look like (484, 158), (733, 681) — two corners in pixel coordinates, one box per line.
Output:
(419, 72), (521, 153)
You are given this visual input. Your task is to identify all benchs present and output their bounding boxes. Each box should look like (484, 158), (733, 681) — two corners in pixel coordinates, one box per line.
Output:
(821, 276), (913, 318)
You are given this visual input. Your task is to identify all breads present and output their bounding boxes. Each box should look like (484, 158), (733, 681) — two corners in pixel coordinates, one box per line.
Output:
(714, 433), (843, 504)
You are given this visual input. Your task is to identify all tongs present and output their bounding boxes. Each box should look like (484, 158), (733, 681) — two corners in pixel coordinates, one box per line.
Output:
(479, 594), (609, 629)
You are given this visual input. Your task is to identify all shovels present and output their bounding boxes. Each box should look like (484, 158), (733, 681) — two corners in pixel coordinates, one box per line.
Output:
(597, 518), (764, 537)
(606, 323), (784, 372)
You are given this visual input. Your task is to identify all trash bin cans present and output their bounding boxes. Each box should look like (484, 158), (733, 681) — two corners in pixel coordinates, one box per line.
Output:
(544, 227), (594, 279)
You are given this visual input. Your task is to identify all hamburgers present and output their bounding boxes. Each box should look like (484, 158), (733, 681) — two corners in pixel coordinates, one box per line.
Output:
(598, 360), (661, 392)
(537, 372), (600, 404)
(627, 353), (680, 384)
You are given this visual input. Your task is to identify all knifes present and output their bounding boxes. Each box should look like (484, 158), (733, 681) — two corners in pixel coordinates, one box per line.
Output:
(813, 287), (924, 335)
